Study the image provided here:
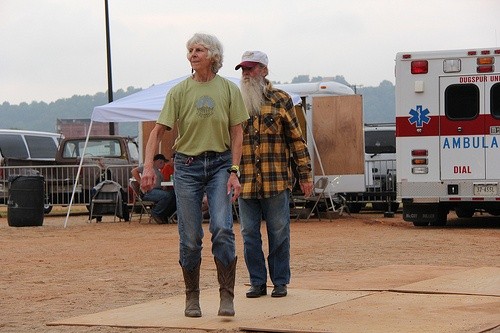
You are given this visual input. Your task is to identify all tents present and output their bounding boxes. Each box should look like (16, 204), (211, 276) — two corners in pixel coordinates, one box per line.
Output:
(64, 74), (334, 227)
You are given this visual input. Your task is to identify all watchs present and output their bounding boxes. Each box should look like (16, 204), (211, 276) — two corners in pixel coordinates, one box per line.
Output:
(228, 169), (240, 178)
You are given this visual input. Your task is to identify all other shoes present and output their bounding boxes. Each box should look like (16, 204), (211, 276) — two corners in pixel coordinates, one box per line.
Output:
(152, 214), (168, 224)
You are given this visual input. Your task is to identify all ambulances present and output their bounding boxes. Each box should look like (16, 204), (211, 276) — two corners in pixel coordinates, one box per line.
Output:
(393, 47), (500, 228)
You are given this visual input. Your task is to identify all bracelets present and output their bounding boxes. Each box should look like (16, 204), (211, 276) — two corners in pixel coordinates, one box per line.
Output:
(231, 166), (239, 170)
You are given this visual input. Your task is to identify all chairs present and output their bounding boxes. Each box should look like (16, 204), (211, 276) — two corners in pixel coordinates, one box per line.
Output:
(128, 181), (156, 224)
(293, 177), (333, 223)
(88, 180), (124, 224)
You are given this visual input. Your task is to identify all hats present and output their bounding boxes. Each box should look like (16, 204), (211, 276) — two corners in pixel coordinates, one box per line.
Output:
(235, 50), (268, 70)
(154, 154), (169, 162)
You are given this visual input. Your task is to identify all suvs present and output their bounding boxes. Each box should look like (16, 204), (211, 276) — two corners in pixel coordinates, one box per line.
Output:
(0, 129), (73, 205)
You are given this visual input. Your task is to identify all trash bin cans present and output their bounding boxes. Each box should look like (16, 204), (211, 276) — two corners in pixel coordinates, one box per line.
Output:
(7, 175), (46, 227)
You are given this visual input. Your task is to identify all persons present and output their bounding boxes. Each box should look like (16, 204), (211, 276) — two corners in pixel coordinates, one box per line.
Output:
(130, 154), (171, 223)
(235, 51), (315, 298)
(141, 33), (250, 317)
(161, 154), (175, 222)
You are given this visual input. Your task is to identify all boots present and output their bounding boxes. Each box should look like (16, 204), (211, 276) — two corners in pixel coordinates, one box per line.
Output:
(178, 257), (202, 317)
(214, 255), (238, 316)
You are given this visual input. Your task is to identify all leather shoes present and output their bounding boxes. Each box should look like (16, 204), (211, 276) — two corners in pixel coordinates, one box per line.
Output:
(271, 285), (288, 297)
(246, 289), (266, 298)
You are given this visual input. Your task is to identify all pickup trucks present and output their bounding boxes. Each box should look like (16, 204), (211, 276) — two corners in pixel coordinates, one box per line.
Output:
(0, 134), (139, 215)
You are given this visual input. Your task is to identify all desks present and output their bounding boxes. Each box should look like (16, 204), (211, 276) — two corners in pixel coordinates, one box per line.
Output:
(160, 181), (178, 224)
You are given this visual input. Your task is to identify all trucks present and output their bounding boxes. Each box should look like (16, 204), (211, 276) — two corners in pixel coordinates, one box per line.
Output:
(138, 80), (368, 213)
(340, 122), (401, 214)
